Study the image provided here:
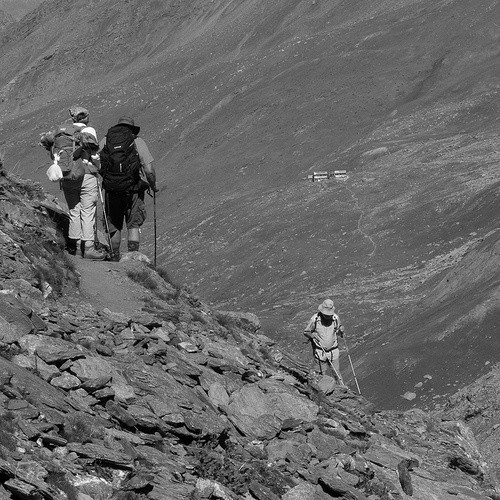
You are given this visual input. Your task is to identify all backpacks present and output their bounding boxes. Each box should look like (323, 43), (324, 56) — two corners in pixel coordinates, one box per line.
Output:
(50, 124), (98, 182)
(99, 124), (150, 193)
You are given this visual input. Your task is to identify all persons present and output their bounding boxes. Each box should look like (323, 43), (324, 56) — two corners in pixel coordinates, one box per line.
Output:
(304, 299), (345, 386)
(41, 106), (104, 260)
(96, 115), (156, 262)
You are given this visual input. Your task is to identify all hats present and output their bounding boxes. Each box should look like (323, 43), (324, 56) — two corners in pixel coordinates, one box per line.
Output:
(69, 108), (90, 124)
(118, 116), (134, 126)
(318, 299), (336, 316)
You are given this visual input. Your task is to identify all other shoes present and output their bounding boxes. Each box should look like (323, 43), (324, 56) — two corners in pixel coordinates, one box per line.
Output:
(110, 248), (120, 262)
(75, 246), (106, 262)
